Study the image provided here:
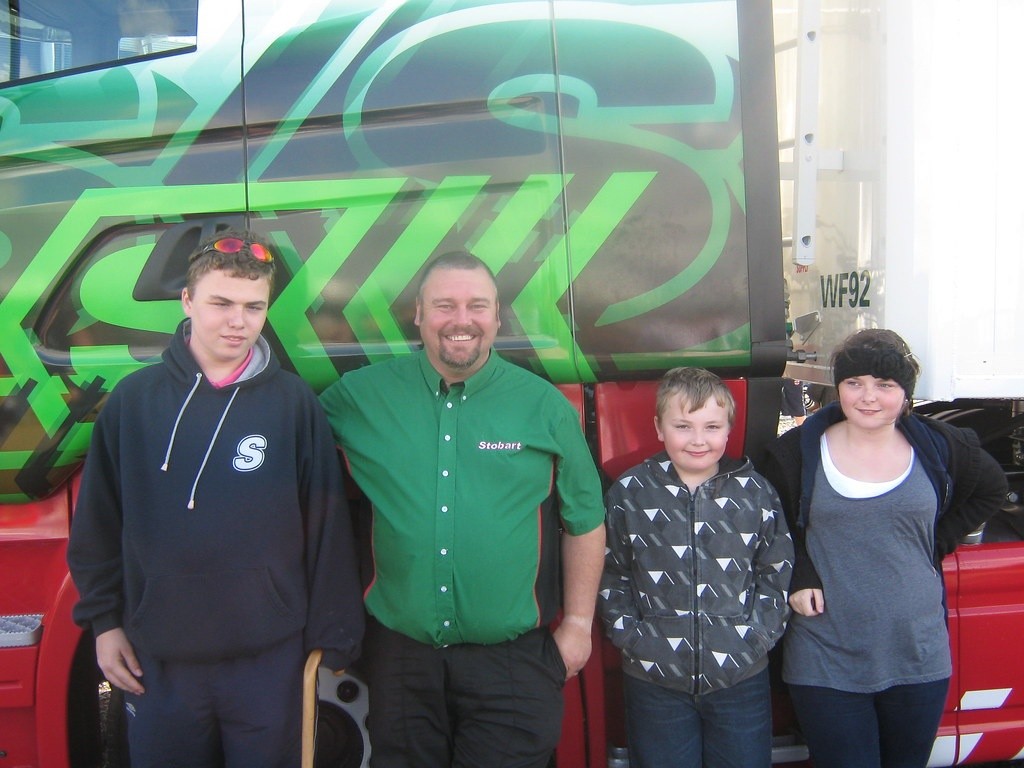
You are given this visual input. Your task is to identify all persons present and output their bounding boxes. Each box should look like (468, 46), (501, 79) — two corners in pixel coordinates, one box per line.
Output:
(65, 229), (365, 768)
(757, 329), (1009, 767)
(597, 368), (794, 768)
(318, 252), (607, 768)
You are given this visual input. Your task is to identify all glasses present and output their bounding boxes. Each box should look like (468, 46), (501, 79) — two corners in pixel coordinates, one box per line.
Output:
(189, 237), (278, 266)
